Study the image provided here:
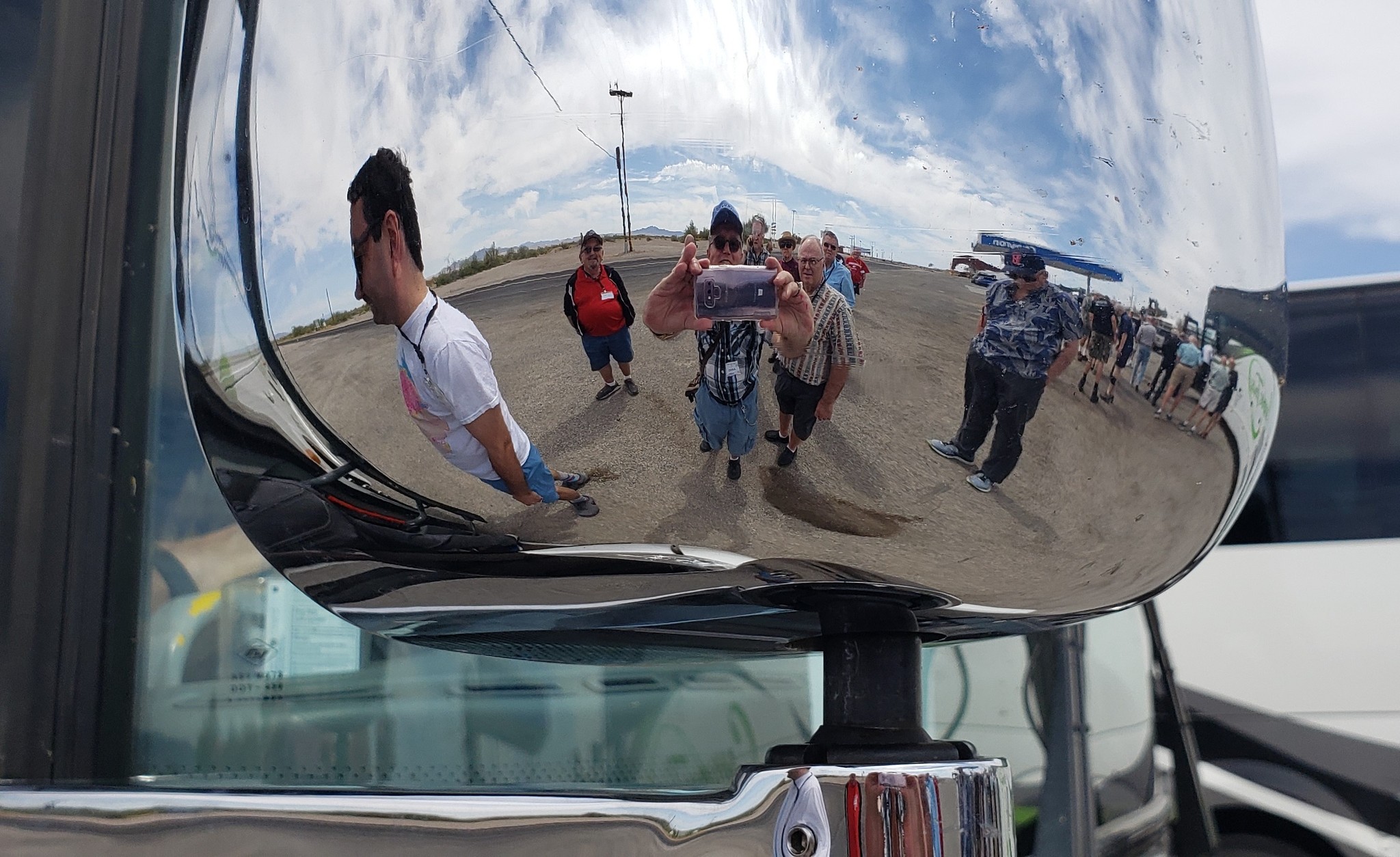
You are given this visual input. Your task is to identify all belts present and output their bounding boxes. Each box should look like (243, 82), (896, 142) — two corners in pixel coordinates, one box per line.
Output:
(705, 379), (756, 406)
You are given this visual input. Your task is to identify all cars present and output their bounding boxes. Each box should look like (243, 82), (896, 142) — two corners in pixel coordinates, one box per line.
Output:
(957, 271), (999, 287)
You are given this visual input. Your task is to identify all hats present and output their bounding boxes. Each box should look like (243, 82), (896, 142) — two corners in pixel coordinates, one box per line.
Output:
(581, 229), (603, 245)
(712, 200), (744, 236)
(1004, 248), (1045, 270)
(778, 231), (796, 251)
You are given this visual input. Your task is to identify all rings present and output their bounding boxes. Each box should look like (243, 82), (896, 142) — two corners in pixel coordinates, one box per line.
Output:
(793, 282), (801, 298)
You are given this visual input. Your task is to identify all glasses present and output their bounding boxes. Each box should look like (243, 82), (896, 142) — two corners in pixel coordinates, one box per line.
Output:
(822, 242), (837, 251)
(351, 215), (400, 289)
(580, 246), (602, 255)
(1004, 270), (1048, 282)
(780, 242), (793, 249)
(798, 257), (824, 266)
(708, 235), (744, 254)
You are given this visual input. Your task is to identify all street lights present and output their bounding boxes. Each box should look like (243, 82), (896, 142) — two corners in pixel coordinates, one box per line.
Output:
(608, 81), (634, 252)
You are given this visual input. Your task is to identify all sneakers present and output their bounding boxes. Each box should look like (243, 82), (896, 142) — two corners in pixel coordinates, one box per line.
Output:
(930, 439), (975, 465)
(595, 380), (620, 399)
(966, 470), (996, 493)
(568, 494), (599, 517)
(624, 378), (639, 394)
(562, 473), (589, 491)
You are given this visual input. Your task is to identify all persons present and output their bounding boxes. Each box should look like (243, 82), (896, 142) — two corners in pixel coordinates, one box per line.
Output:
(1077, 292), (1238, 439)
(846, 248), (870, 296)
(768, 232), (801, 373)
(741, 215), (772, 266)
(765, 235), (866, 467)
(563, 229), (639, 400)
(642, 202), (814, 480)
(930, 250), (1084, 493)
(346, 148), (600, 523)
(821, 231), (855, 308)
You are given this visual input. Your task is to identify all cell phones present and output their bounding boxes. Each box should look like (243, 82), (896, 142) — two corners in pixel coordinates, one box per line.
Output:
(693, 263), (778, 322)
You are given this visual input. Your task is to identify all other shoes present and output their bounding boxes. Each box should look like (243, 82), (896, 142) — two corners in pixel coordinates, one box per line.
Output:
(778, 446), (798, 466)
(1078, 351), (1209, 439)
(699, 439), (712, 451)
(764, 430), (790, 443)
(728, 455), (741, 479)
